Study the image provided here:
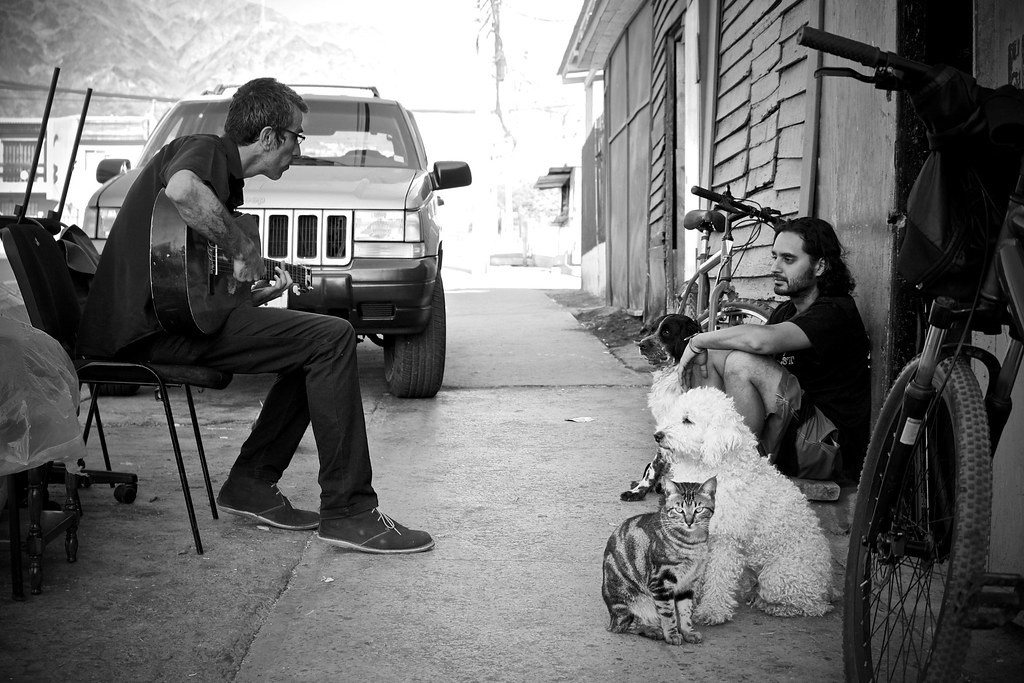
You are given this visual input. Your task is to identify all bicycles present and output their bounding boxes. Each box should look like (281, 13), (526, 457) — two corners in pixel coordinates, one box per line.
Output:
(632, 181), (787, 369)
(794, 19), (1023, 682)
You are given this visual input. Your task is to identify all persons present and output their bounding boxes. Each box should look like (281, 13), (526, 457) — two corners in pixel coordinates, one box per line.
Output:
(678, 216), (873, 487)
(79, 78), (435, 555)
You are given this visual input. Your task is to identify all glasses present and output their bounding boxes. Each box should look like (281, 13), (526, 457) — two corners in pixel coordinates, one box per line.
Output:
(275, 127), (305, 145)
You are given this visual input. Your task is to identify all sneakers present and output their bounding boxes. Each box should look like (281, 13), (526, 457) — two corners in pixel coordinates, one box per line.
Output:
(318, 507), (434, 551)
(216, 479), (319, 529)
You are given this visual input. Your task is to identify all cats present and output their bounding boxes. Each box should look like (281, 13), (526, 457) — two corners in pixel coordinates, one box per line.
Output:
(600, 475), (718, 646)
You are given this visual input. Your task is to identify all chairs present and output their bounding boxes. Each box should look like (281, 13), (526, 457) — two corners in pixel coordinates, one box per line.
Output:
(0, 218), (233, 555)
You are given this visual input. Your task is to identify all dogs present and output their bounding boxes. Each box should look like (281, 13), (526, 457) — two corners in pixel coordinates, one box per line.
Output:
(654, 384), (835, 626)
(620, 314), (704, 502)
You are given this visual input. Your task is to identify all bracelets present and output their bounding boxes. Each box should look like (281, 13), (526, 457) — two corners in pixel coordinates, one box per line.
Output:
(689, 333), (706, 355)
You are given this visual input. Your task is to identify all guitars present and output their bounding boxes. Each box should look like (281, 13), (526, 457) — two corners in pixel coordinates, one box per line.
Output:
(149, 186), (313, 339)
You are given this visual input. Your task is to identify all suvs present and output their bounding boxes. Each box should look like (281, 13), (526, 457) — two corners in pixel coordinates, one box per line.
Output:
(80, 82), (473, 399)
